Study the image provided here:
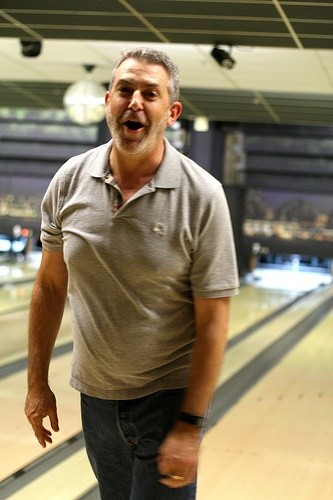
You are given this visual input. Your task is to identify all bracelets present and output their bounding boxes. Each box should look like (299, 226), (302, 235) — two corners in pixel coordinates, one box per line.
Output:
(175, 410), (208, 429)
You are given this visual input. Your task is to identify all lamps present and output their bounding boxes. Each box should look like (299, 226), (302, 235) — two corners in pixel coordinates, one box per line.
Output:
(211, 44), (237, 69)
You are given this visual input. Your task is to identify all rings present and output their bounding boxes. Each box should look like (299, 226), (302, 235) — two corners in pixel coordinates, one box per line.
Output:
(172, 475), (184, 481)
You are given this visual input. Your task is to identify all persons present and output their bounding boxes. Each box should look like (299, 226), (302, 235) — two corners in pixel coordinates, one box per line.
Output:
(23, 47), (240, 500)
